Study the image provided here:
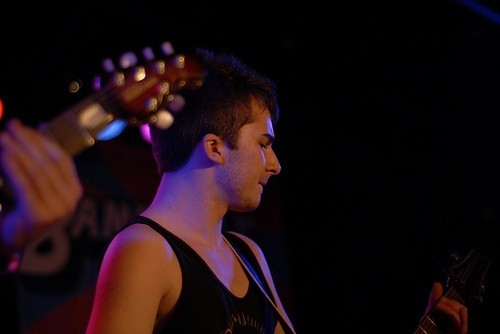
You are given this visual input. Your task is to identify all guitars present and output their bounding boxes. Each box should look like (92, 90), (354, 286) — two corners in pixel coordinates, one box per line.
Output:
(411, 247), (492, 334)
(32, 38), (206, 158)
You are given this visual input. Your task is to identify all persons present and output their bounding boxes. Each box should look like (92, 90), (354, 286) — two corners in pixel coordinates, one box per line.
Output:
(85, 52), (468, 334)
(0, 100), (83, 280)
